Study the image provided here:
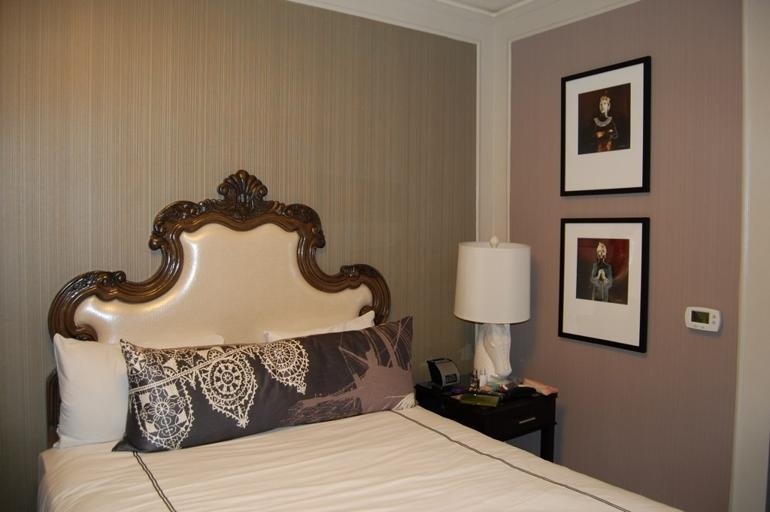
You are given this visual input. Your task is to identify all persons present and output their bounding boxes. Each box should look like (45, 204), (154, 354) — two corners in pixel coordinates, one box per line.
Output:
(589, 242), (613, 301)
(591, 95), (619, 152)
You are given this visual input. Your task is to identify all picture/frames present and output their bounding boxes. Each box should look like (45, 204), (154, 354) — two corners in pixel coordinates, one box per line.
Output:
(556, 216), (651, 352)
(560, 55), (652, 195)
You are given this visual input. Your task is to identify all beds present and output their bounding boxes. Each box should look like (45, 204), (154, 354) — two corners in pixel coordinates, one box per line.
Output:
(35, 170), (685, 510)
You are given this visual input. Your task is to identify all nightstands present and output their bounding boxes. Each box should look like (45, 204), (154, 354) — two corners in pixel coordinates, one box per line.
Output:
(412, 376), (560, 464)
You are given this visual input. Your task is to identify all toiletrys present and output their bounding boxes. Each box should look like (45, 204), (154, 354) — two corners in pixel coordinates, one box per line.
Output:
(478, 368), (488, 389)
(470, 368), (478, 388)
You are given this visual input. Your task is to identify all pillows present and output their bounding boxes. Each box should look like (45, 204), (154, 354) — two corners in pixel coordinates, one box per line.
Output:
(257, 307), (379, 344)
(109, 313), (420, 451)
(49, 328), (224, 450)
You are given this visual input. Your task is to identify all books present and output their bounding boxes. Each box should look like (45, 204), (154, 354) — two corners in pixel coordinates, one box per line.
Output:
(461, 394), (500, 408)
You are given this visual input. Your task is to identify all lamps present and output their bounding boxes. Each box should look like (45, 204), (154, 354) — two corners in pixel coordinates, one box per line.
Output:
(453, 235), (532, 390)
